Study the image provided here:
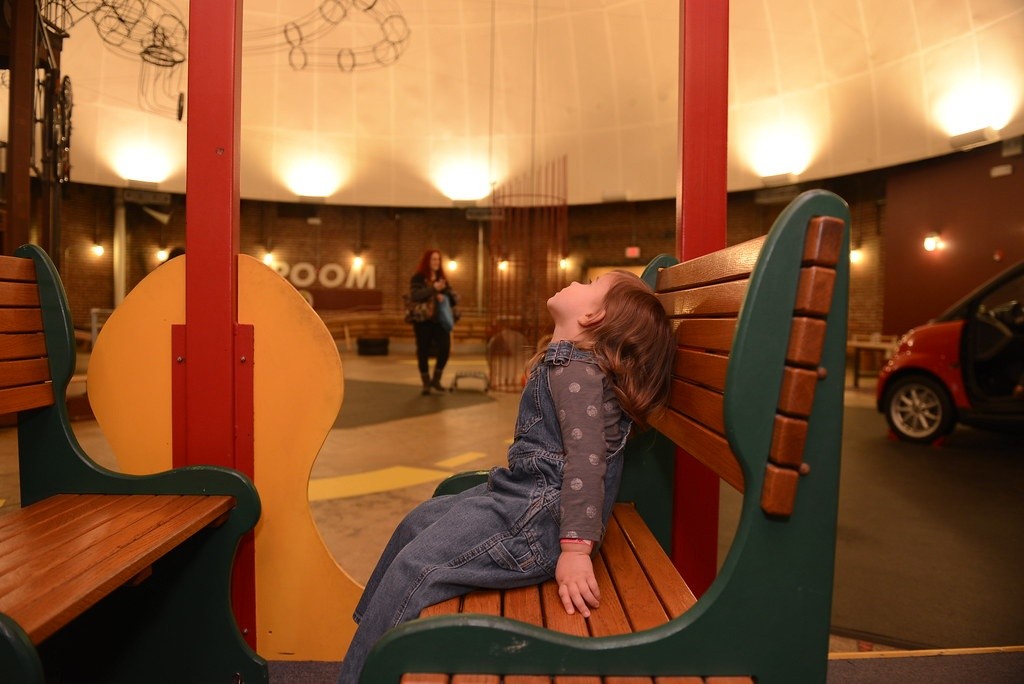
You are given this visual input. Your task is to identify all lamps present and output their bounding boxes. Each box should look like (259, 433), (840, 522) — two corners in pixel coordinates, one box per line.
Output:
(126, 179), (158, 192)
(602, 189), (630, 202)
(299, 196), (326, 204)
(453, 200), (477, 208)
(762, 173), (798, 188)
(949, 125), (1000, 149)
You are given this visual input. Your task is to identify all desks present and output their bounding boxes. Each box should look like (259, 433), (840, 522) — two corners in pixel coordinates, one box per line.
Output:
(847, 341), (892, 388)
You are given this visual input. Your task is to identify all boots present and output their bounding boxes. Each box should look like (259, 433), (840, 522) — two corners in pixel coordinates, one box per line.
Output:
(432, 367), (445, 391)
(420, 372), (430, 394)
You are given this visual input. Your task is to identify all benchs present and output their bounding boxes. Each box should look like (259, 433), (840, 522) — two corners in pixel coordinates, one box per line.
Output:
(362, 189), (850, 684)
(0, 244), (267, 684)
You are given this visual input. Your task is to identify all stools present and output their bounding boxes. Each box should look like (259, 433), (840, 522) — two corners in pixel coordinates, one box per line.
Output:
(450, 370), (489, 393)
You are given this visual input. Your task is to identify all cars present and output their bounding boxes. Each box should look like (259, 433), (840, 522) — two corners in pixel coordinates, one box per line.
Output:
(874, 256), (1024, 446)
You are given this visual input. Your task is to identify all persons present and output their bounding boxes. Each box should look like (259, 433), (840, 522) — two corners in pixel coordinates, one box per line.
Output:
(410, 250), (456, 396)
(339, 270), (672, 684)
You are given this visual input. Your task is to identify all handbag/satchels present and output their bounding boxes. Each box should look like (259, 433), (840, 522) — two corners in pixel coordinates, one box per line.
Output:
(437, 294), (452, 330)
(405, 302), (435, 322)
